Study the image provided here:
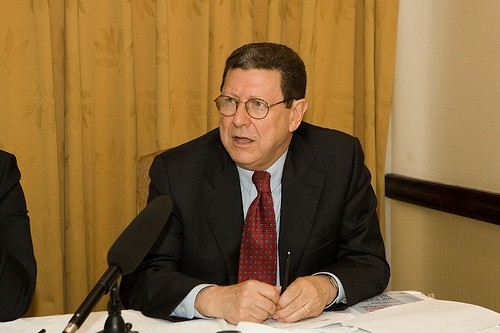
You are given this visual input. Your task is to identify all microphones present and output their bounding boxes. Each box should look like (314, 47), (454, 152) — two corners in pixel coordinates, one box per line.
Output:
(62, 195), (173, 333)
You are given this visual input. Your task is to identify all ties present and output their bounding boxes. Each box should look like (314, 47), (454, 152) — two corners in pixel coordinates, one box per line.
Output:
(237, 171), (277, 286)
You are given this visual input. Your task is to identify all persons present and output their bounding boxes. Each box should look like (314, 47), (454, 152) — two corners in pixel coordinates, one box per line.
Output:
(119, 42), (390, 326)
(0, 150), (37, 322)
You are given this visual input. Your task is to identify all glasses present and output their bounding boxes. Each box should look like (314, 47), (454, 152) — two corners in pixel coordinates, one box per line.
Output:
(214, 94), (298, 120)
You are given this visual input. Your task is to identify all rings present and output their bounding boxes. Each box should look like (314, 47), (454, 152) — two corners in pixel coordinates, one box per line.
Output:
(303, 306), (310, 314)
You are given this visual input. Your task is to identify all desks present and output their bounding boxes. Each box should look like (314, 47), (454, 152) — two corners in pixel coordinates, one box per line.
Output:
(0, 290), (500, 333)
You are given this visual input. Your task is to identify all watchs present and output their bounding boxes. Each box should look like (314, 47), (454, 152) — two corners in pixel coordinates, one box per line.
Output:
(314, 274), (339, 307)
